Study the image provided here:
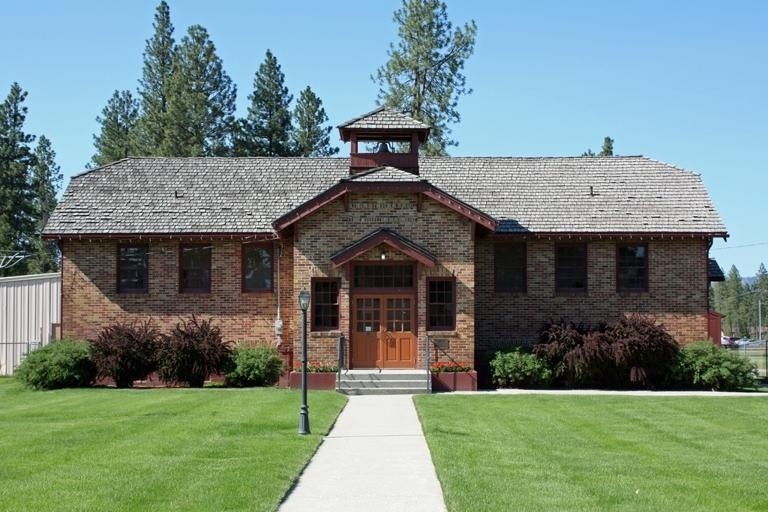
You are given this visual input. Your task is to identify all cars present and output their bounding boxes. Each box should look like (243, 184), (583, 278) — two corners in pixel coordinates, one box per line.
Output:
(720, 329), (767, 349)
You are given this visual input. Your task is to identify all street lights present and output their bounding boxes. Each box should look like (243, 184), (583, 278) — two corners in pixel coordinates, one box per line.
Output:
(297, 286), (312, 435)
